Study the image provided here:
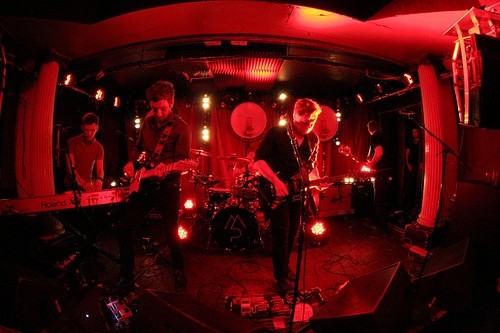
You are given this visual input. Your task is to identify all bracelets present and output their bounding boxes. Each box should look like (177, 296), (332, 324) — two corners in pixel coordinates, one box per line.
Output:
(95, 177), (103, 182)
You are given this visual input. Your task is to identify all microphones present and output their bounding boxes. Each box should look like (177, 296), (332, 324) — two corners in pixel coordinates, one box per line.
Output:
(399, 109), (415, 116)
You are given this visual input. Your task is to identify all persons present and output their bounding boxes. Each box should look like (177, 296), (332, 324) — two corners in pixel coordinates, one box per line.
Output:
(366, 120), (423, 238)
(39, 112), (105, 239)
(254, 98), (322, 295)
(118, 81), (189, 298)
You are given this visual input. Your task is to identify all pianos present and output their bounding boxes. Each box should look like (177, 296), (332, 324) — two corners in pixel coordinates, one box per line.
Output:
(0, 187), (127, 254)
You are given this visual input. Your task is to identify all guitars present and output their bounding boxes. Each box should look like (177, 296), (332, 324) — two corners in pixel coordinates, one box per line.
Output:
(125, 159), (199, 198)
(338, 145), (380, 179)
(257, 171), (358, 209)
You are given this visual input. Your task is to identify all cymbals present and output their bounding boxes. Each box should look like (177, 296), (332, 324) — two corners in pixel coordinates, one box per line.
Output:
(191, 148), (217, 157)
(216, 155), (251, 169)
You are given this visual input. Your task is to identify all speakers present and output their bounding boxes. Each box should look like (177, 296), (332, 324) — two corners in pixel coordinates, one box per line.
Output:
(457, 122), (500, 185)
(135, 287), (275, 333)
(318, 183), (375, 219)
(411, 238), (475, 311)
(310, 262), (417, 333)
(444, 181), (500, 284)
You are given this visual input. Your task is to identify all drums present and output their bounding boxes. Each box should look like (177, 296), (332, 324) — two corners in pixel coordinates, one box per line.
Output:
(191, 172), (270, 252)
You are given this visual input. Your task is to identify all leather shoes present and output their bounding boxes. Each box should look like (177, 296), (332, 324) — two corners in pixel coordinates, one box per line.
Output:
(277, 271), (296, 292)
(173, 268), (186, 292)
